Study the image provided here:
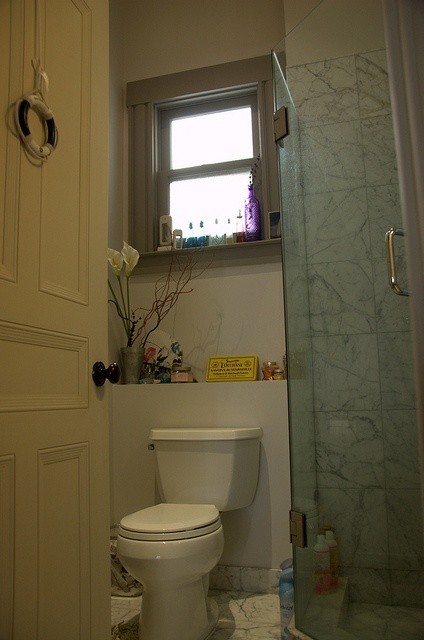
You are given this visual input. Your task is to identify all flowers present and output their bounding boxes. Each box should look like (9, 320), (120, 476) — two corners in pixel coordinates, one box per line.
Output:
(108, 225), (223, 347)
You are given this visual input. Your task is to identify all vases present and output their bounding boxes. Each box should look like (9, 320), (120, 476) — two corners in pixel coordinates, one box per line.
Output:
(119, 345), (146, 386)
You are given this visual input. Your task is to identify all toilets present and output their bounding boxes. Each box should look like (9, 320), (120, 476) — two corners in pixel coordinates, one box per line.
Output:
(116, 428), (261, 640)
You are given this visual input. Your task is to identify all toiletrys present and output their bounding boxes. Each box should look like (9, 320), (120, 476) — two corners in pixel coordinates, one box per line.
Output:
(171, 367), (194, 382)
